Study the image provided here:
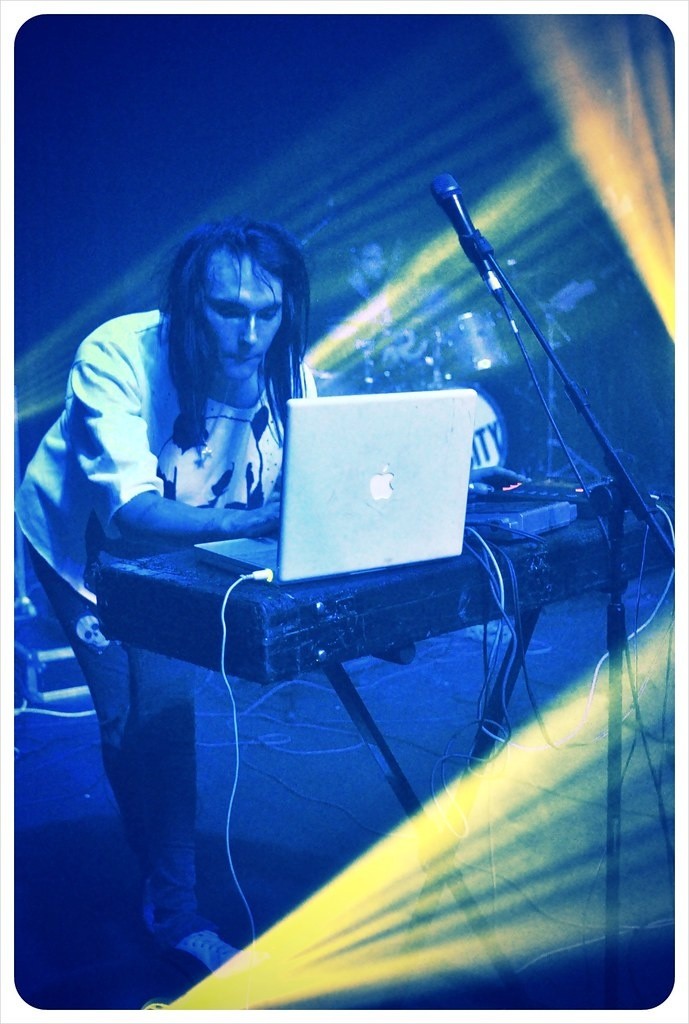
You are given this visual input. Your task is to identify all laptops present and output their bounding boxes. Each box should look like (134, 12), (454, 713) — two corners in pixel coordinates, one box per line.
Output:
(193, 388), (477, 583)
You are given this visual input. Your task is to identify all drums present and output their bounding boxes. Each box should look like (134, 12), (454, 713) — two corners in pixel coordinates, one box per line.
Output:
(383, 383), (508, 469)
(431, 310), (507, 379)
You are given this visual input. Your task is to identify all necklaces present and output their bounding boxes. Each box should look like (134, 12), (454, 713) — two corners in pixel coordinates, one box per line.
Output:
(193, 377), (232, 459)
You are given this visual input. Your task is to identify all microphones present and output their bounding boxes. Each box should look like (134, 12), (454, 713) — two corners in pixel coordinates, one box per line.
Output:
(430, 172), (506, 306)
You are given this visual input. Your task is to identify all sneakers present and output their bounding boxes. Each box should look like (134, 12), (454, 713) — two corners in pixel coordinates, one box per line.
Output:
(142, 874), (239, 985)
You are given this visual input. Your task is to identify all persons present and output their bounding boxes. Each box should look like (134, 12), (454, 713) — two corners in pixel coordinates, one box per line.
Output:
(15, 214), (316, 987)
(320, 240), (383, 376)
